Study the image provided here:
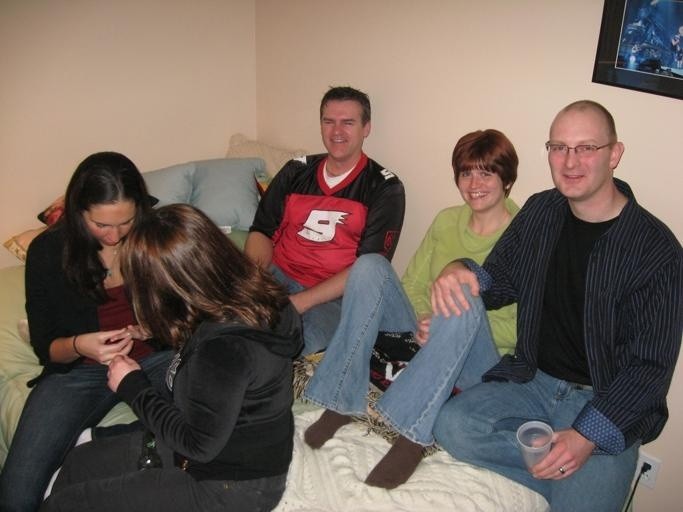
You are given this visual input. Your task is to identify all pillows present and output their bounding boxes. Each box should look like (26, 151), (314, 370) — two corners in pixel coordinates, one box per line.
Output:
(138, 157), (267, 230)
(35, 195), (68, 231)
(223, 133), (307, 181)
(1, 226), (47, 262)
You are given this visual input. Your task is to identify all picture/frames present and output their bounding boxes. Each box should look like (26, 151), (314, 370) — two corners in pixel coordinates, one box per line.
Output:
(590, 1), (683, 101)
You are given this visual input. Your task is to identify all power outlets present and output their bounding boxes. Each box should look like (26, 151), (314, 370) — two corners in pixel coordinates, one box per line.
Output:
(632, 449), (665, 494)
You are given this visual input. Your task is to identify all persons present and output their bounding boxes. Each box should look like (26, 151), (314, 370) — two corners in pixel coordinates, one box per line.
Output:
(39, 203), (305, 512)
(0, 152), (176, 511)
(432, 100), (683, 512)
(299, 129), (520, 489)
(243, 85), (404, 355)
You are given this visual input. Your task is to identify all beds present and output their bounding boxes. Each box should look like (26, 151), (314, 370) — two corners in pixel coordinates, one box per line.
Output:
(0, 196), (569, 510)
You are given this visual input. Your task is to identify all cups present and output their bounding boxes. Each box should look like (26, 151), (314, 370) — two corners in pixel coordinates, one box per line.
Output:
(515, 419), (554, 474)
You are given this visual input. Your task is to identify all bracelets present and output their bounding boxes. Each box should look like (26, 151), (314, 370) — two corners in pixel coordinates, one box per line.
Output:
(72, 335), (82, 357)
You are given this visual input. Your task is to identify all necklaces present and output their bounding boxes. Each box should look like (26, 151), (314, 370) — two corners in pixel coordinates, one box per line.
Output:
(97, 241), (120, 277)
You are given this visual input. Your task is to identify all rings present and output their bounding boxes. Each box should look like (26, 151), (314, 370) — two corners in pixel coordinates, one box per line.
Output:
(559, 467), (565, 474)
(125, 330), (133, 336)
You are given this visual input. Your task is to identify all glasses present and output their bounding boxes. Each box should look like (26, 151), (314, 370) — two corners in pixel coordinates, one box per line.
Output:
(544, 140), (616, 158)
